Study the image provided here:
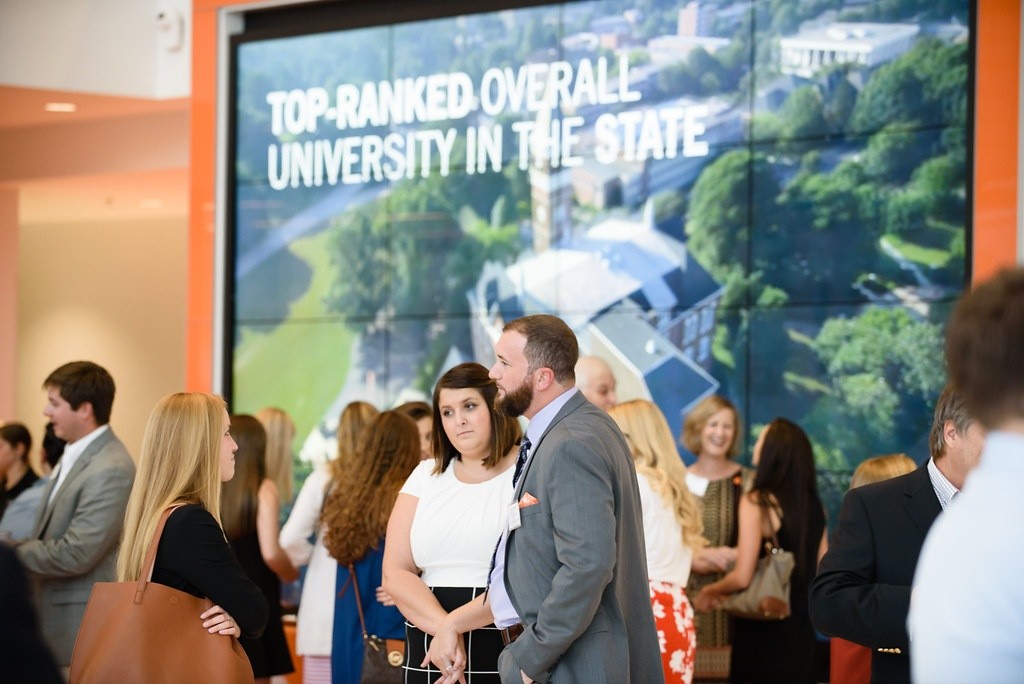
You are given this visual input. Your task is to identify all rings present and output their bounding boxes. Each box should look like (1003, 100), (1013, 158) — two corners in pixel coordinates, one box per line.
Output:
(446, 665), (452, 670)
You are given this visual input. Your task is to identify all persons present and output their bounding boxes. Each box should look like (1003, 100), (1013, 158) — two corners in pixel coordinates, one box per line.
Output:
(278, 402), (380, 684)
(818, 452), (919, 684)
(396, 401), (436, 467)
(376, 362), (530, 684)
(0, 360), (136, 684)
(699, 418), (827, 684)
(905, 269), (1023, 684)
(808, 382), (989, 684)
(0, 423), (39, 518)
(604, 399), (708, 684)
(0, 540), (67, 684)
(219, 415), (299, 684)
(253, 405), (297, 506)
(321, 410), (422, 684)
(572, 355), (619, 410)
(680, 393), (755, 684)
(0, 422), (68, 532)
(427, 314), (663, 684)
(118, 391), (269, 637)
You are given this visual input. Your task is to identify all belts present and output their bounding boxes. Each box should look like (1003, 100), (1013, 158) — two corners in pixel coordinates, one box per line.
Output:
(500, 624), (525, 647)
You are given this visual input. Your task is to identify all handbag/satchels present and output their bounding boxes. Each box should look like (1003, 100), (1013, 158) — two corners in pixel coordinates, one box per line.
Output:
(359, 636), (407, 684)
(711, 495), (795, 620)
(68, 504), (255, 684)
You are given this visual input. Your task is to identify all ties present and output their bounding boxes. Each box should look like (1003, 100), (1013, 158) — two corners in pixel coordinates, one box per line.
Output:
(484, 434), (532, 605)
